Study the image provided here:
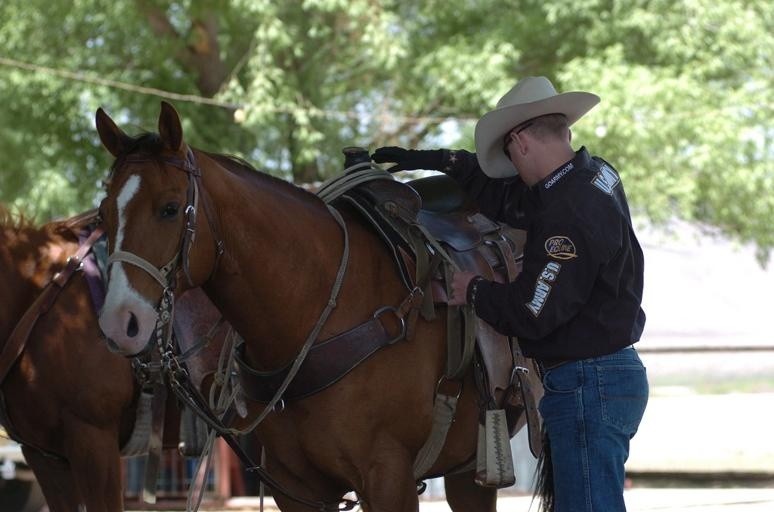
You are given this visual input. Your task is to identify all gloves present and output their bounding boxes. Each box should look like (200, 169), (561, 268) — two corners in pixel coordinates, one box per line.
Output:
(370, 145), (444, 175)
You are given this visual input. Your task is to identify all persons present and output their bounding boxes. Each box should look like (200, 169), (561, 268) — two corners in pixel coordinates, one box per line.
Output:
(372, 76), (650, 511)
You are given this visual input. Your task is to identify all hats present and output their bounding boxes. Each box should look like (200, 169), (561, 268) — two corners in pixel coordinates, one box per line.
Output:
(474, 76), (601, 179)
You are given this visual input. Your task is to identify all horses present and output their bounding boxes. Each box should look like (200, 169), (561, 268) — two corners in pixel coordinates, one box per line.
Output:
(0, 197), (231, 512)
(96, 99), (553, 512)
